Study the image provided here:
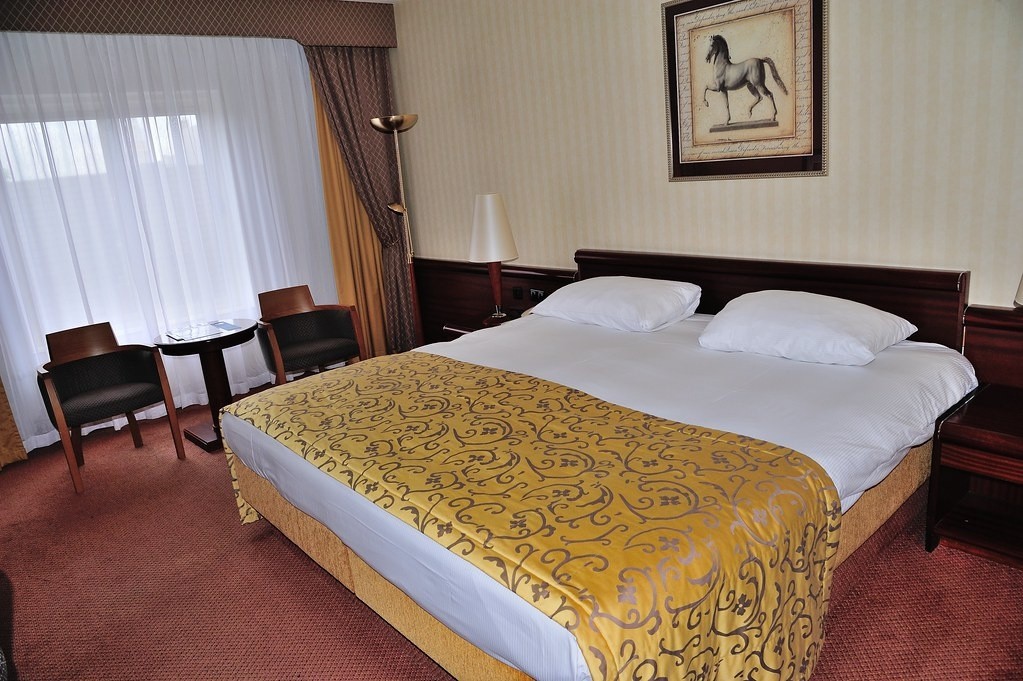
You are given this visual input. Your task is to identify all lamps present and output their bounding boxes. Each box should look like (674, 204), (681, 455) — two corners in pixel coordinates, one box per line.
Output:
(467, 193), (519, 326)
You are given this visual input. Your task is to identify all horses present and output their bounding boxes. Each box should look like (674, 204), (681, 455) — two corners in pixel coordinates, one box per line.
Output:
(703, 35), (788, 126)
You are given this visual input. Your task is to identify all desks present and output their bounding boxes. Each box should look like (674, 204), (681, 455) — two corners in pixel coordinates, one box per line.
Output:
(154, 319), (258, 453)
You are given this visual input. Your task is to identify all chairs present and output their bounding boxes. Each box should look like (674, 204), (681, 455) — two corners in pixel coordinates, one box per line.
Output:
(36, 321), (186, 495)
(257, 284), (368, 385)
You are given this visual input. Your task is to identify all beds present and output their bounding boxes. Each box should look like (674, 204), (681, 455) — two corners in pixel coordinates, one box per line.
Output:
(219, 249), (979, 681)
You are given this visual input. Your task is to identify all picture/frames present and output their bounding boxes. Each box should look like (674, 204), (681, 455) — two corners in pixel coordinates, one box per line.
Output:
(661, 0), (830, 182)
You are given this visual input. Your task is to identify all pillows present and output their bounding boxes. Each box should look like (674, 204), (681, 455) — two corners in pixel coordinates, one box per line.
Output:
(698, 289), (918, 365)
(521, 276), (703, 333)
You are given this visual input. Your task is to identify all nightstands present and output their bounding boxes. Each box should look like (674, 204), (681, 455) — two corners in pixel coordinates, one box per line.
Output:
(925, 381), (1023, 570)
(442, 310), (526, 340)
